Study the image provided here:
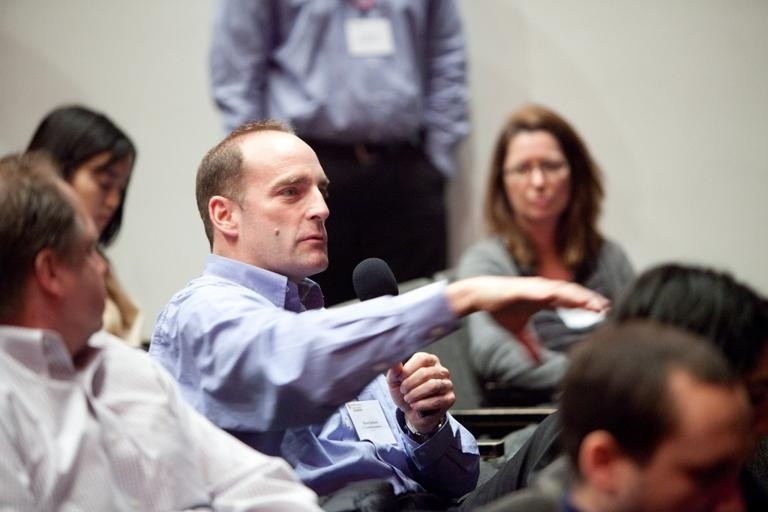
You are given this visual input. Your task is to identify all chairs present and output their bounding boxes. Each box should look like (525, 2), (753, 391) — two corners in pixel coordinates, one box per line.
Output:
(324, 266), (560, 458)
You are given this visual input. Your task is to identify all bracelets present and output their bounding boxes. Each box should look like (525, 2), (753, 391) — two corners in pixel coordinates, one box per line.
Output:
(400, 414), (443, 443)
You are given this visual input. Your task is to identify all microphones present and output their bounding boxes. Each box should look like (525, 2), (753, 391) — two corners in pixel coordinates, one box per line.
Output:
(352, 257), (436, 420)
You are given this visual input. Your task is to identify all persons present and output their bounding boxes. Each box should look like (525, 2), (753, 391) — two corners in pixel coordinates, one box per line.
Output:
(458, 261), (768, 510)
(471, 318), (756, 511)
(22, 100), (151, 348)
(0, 148), (324, 510)
(206, 1), (474, 309)
(454, 104), (642, 452)
(151, 117), (614, 511)
